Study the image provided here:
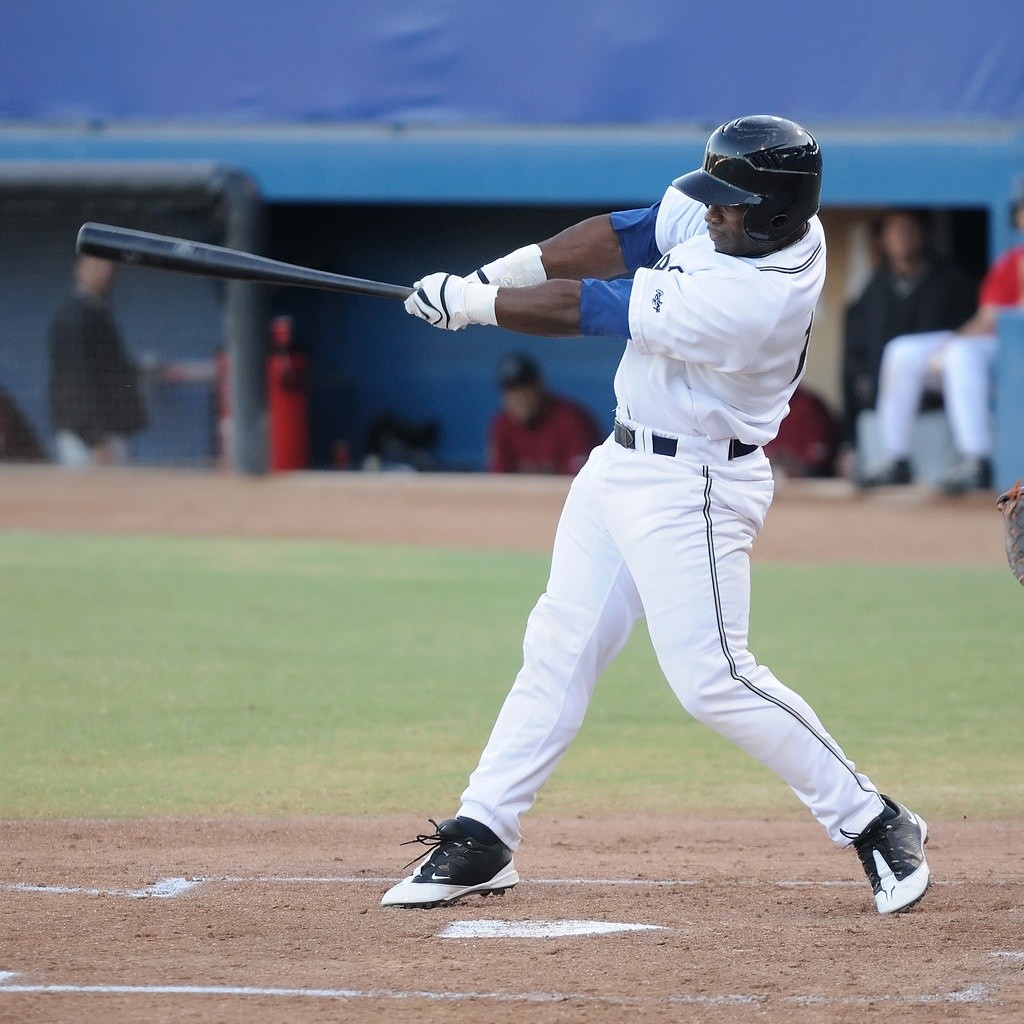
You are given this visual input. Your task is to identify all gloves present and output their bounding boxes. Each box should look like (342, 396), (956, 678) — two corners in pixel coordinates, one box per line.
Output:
(463, 244), (547, 287)
(404, 271), (499, 331)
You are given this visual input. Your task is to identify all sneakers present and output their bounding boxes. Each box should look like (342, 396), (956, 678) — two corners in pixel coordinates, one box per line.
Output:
(381, 818), (520, 909)
(840, 794), (931, 916)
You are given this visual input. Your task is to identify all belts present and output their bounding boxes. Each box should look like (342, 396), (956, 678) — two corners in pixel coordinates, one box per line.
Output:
(614, 417), (758, 461)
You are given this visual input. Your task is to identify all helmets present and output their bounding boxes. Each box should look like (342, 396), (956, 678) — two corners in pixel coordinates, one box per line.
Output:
(671, 115), (822, 242)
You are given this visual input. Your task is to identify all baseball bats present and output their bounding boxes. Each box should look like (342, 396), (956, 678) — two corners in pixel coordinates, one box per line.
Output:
(74, 220), (416, 299)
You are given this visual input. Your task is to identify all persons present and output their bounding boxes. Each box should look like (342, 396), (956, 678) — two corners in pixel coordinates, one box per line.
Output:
(766, 175), (1023, 498)
(381, 114), (929, 916)
(47, 256), (314, 472)
(484, 352), (604, 477)
